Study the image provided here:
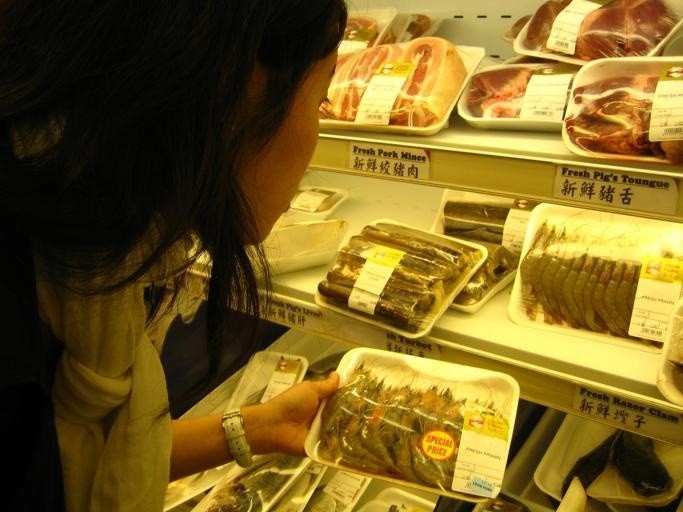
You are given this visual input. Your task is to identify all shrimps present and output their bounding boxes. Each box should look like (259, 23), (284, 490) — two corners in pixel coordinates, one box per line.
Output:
(519, 218), (638, 340)
(315, 361), (467, 494)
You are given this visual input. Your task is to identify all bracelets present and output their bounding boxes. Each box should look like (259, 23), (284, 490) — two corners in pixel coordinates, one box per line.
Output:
(216, 407), (256, 471)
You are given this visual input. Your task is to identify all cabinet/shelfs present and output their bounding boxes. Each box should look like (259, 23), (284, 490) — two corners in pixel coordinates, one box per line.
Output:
(166, 129), (683, 512)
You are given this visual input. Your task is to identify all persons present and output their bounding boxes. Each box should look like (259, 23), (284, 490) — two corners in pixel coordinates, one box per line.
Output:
(0, 1), (350, 512)
